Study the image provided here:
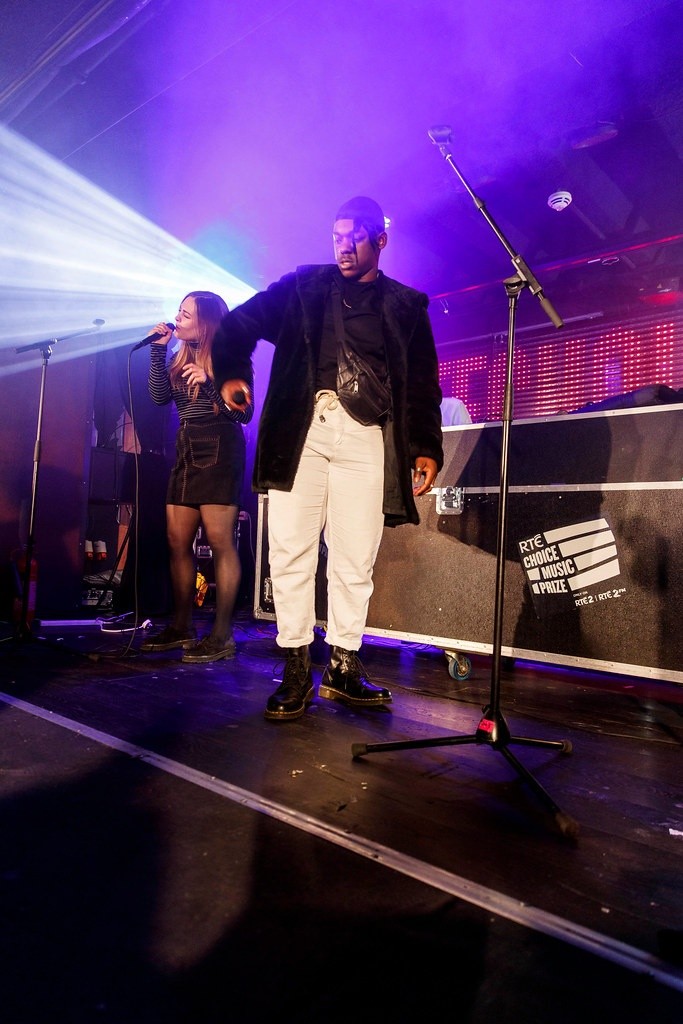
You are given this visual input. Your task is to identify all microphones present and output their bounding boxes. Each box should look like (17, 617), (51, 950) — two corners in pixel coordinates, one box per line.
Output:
(232, 391), (245, 405)
(132, 323), (175, 351)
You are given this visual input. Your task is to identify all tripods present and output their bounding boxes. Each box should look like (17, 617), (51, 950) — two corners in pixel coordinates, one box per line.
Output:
(0, 319), (105, 666)
(351, 125), (582, 839)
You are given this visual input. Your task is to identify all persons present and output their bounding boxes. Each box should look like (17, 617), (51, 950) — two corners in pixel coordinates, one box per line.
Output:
(210, 196), (443, 722)
(139, 291), (247, 664)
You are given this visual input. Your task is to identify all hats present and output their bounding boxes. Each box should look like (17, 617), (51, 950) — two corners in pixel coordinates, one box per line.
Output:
(335, 195), (386, 231)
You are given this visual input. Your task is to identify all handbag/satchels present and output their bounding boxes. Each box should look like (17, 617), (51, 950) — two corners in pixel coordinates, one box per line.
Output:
(334, 337), (393, 428)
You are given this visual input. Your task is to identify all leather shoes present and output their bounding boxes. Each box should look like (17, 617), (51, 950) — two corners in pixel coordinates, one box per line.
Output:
(316, 643), (394, 707)
(181, 636), (237, 663)
(139, 629), (199, 651)
(264, 645), (317, 721)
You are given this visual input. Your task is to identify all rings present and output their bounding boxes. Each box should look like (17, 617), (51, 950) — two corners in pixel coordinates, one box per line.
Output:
(415, 467), (421, 472)
